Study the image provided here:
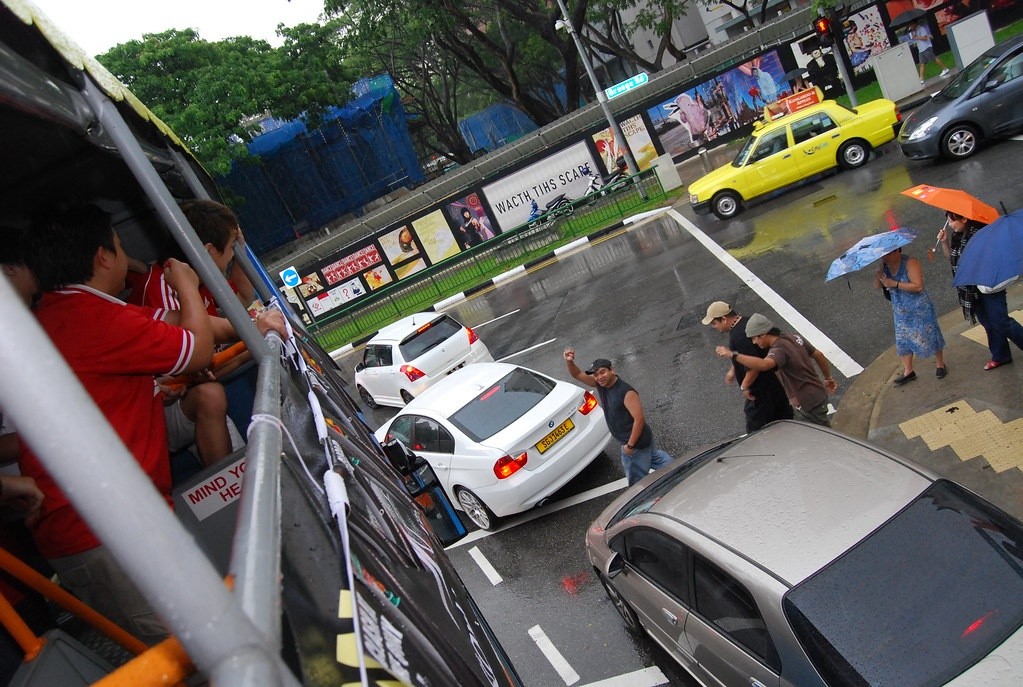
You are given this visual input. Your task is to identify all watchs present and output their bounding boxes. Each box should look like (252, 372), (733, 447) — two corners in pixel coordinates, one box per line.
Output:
(741, 386), (748, 391)
(732, 351), (738, 362)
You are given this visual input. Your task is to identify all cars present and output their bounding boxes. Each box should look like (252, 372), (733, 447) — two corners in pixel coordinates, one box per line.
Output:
(373, 362), (613, 532)
(687, 86), (904, 220)
(585, 417), (1023, 687)
(354, 311), (496, 412)
(897, 32), (1023, 164)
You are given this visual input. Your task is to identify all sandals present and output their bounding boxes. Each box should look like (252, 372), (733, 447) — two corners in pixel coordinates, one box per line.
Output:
(984, 357), (1012, 370)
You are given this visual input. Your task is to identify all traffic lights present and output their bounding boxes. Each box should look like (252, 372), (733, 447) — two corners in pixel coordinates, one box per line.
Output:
(812, 15), (836, 49)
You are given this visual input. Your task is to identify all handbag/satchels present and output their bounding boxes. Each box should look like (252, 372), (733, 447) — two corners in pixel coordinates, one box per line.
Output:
(977, 274), (1019, 295)
(881, 262), (892, 301)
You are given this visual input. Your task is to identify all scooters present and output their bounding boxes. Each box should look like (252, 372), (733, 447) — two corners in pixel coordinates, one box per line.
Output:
(583, 155), (632, 207)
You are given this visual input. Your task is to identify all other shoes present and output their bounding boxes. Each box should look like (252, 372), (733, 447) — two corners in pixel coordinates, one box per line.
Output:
(939, 68), (949, 77)
(894, 370), (916, 384)
(935, 364), (947, 378)
(920, 78), (923, 84)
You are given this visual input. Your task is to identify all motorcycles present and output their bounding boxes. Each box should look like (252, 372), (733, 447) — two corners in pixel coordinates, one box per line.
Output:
(526, 193), (575, 229)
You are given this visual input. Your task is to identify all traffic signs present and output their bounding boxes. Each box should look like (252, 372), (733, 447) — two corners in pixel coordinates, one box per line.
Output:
(605, 72), (649, 100)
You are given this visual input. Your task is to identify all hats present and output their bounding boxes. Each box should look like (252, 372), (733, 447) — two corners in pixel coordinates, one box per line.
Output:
(702, 300), (732, 326)
(585, 358), (612, 375)
(745, 313), (773, 338)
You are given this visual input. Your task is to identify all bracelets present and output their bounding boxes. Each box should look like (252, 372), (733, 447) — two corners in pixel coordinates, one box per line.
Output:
(895, 282), (900, 289)
(823, 376), (832, 380)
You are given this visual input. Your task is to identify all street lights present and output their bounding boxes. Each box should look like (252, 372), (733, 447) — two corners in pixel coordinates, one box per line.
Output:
(555, 20), (650, 200)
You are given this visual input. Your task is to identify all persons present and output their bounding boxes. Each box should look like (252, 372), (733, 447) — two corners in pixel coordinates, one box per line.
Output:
(460, 209), (484, 249)
(874, 247), (947, 384)
(752, 67), (777, 102)
(937, 210), (1023, 369)
(702, 301), (794, 433)
(793, 76), (809, 94)
(716, 314), (837, 427)
(0, 199), (289, 621)
(564, 350), (673, 487)
(910, 22), (949, 83)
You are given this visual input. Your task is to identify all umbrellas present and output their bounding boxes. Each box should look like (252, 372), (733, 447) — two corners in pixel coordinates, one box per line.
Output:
(824, 227), (918, 290)
(778, 68), (809, 84)
(889, 8), (928, 31)
(901, 185), (999, 253)
(952, 201), (1023, 287)
